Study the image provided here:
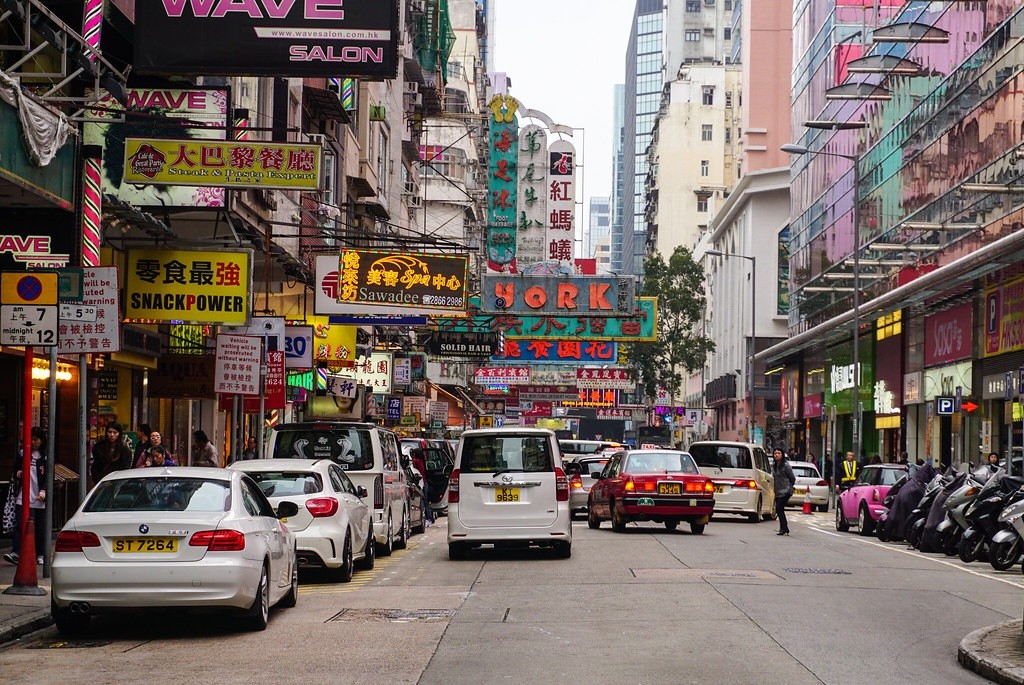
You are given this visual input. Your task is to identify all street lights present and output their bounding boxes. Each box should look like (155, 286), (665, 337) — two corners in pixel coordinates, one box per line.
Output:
(705, 249), (756, 443)
(779, 143), (861, 460)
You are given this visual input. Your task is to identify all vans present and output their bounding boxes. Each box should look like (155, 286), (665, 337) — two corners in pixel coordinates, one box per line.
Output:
(442, 428), (583, 561)
(559, 439), (631, 466)
(274, 423), (460, 557)
(688, 440), (779, 522)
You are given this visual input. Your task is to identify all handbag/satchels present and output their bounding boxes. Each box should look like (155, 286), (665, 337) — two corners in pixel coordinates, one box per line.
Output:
(2, 476), (18, 534)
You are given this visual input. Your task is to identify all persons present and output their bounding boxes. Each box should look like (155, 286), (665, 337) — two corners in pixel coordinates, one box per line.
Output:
(860, 448), (870, 466)
(819, 454), (833, 483)
(136, 430), (174, 469)
(786, 448), (795, 461)
(131, 423), (151, 469)
(91, 422), (132, 486)
(194, 430), (218, 467)
(151, 445), (179, 506)
(986, 452), (999, 473)
(808, 453), (818, 468)
(243, 436), (259, 460)
(870, 455), (882, 485)
(898, 452), (909, 465)
(3, 426), (47, 564)
(772, 448), (795, 536)
(835, 452), (861, 492)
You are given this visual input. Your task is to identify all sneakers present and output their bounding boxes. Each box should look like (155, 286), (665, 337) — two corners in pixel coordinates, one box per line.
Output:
(4, 553), (18, 565)
(36, 557), (44, 565)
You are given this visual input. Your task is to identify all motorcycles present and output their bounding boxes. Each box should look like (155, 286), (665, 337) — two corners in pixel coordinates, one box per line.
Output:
(876, 461), (1024, 572)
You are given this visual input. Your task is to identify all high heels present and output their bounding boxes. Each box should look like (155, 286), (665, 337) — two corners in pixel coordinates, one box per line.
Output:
(777, 527), (789, 536)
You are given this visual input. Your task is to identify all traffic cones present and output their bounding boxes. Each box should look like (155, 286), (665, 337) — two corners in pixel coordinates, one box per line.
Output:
(801, 487), (814, 514)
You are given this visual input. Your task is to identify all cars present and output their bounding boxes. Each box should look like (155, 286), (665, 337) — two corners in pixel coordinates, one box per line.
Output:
(565, 449), (716, 533)
(52, 465), (301, 637)
(834, 463), (909, 537)
(765, 453), (830, 512)
(221, 457), (376, 585)
